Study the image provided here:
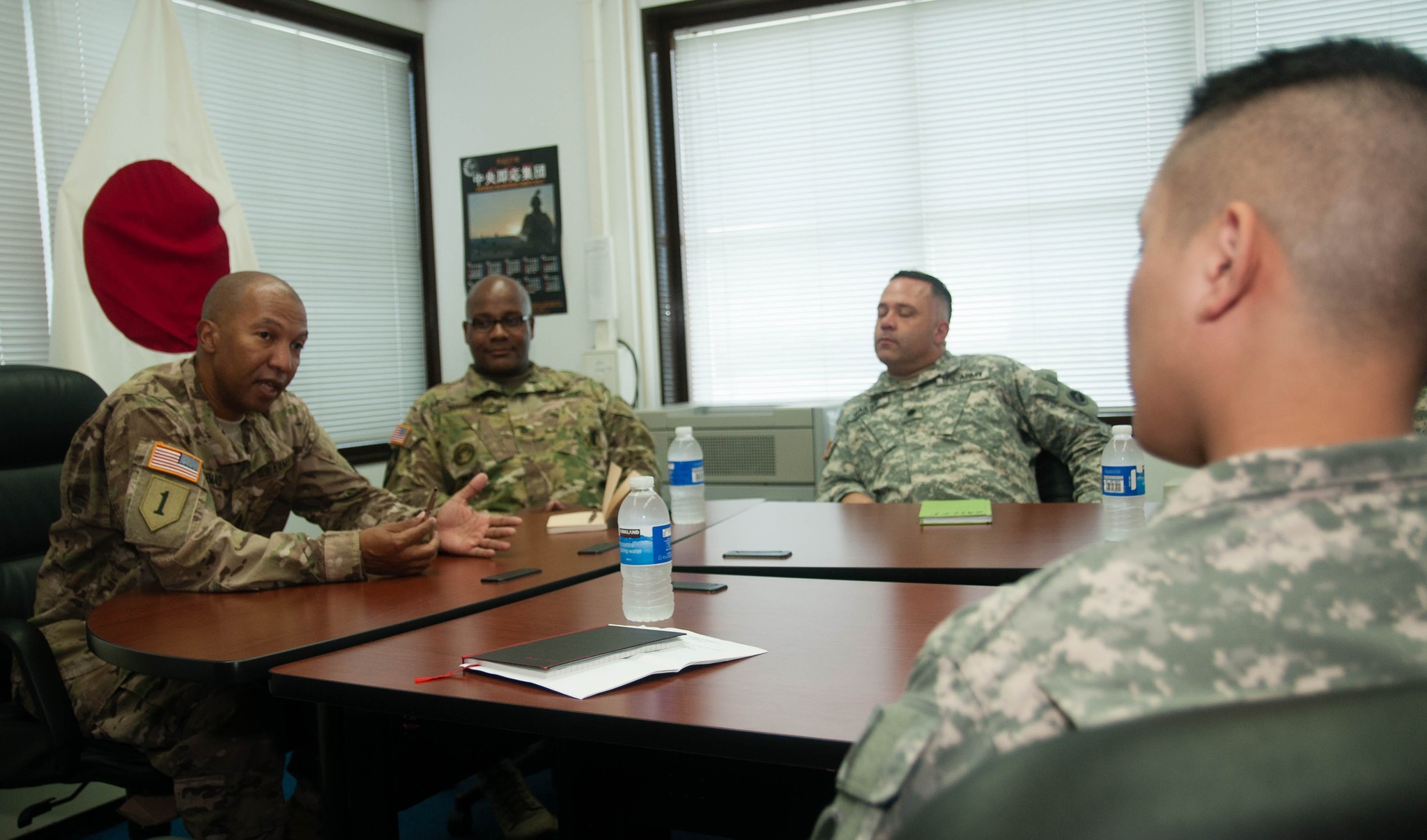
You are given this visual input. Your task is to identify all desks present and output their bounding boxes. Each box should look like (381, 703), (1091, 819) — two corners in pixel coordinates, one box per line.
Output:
(272, 556), (1020, 837)
(661, 499), (1105, 582)
(85, 498), (764, 839)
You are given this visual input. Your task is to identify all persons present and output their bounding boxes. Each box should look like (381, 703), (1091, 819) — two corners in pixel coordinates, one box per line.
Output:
(379, 272), (663, 533)
(820, 28), (1424, 840)
(816, 267), (1118, 508)
(28, 269), (527, 837)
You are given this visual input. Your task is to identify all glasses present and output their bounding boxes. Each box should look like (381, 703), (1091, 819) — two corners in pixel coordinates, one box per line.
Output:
(465, 314), (530, 333)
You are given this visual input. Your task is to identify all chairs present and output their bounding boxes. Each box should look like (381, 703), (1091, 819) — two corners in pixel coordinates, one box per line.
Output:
(1, 362), (175, 840)
(884, 677), (1427, 840)
(1019, 365), (1076, 507)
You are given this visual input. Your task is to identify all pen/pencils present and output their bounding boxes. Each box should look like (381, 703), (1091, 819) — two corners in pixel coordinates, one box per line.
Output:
(421, 486), (438, 523)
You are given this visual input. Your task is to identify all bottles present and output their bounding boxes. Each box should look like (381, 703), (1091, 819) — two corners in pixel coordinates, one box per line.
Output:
(667, 426), (706, 526)
(618, 475), (675, 622)
(1101, 424), (1146, 541)
